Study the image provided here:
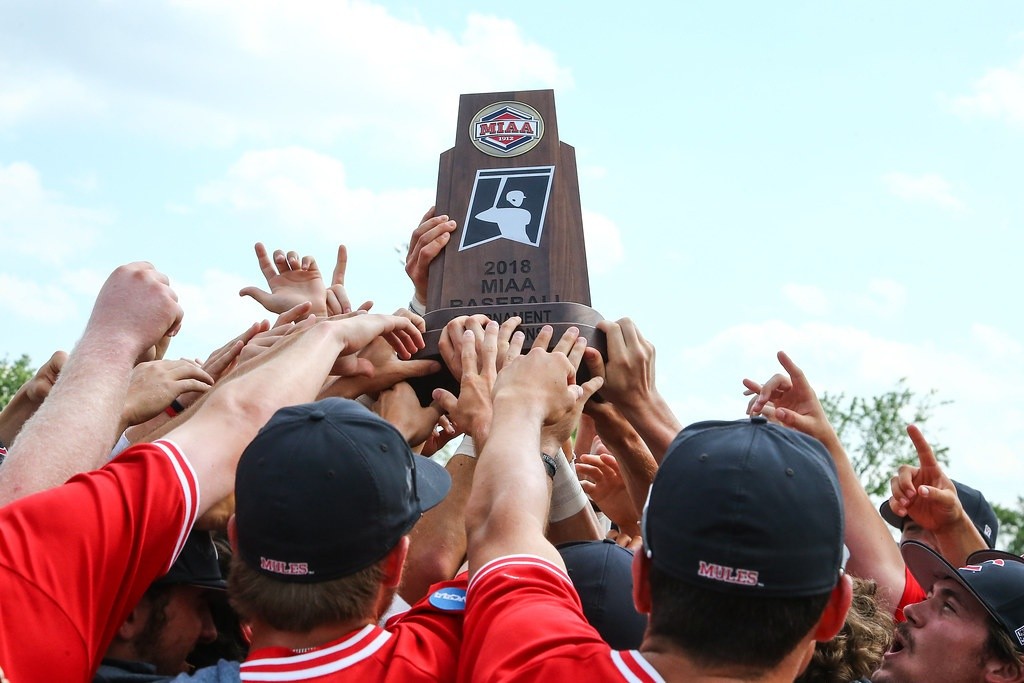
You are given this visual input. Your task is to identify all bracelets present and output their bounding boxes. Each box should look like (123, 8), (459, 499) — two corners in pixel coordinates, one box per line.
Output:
(407, 296), (426, 317)
(541, 452), (557, 479)
(166, 400), (184, 417)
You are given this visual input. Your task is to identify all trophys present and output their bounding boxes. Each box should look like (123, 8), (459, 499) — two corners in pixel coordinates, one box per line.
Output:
(396, 90), (610, 407)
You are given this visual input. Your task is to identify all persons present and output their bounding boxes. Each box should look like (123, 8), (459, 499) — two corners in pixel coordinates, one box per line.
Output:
(0, 208), (1024, 683)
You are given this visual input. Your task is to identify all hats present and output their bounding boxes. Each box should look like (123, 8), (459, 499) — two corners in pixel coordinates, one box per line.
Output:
(640, 416), (851, 598)
(554, 538), (648, 650)
(900, 540), (1024, 652)
(880, 479), (999, 549)
(965, 550), (1024, 564)
(234, 396), (452, 585)
(151, 530), (231, 592)
